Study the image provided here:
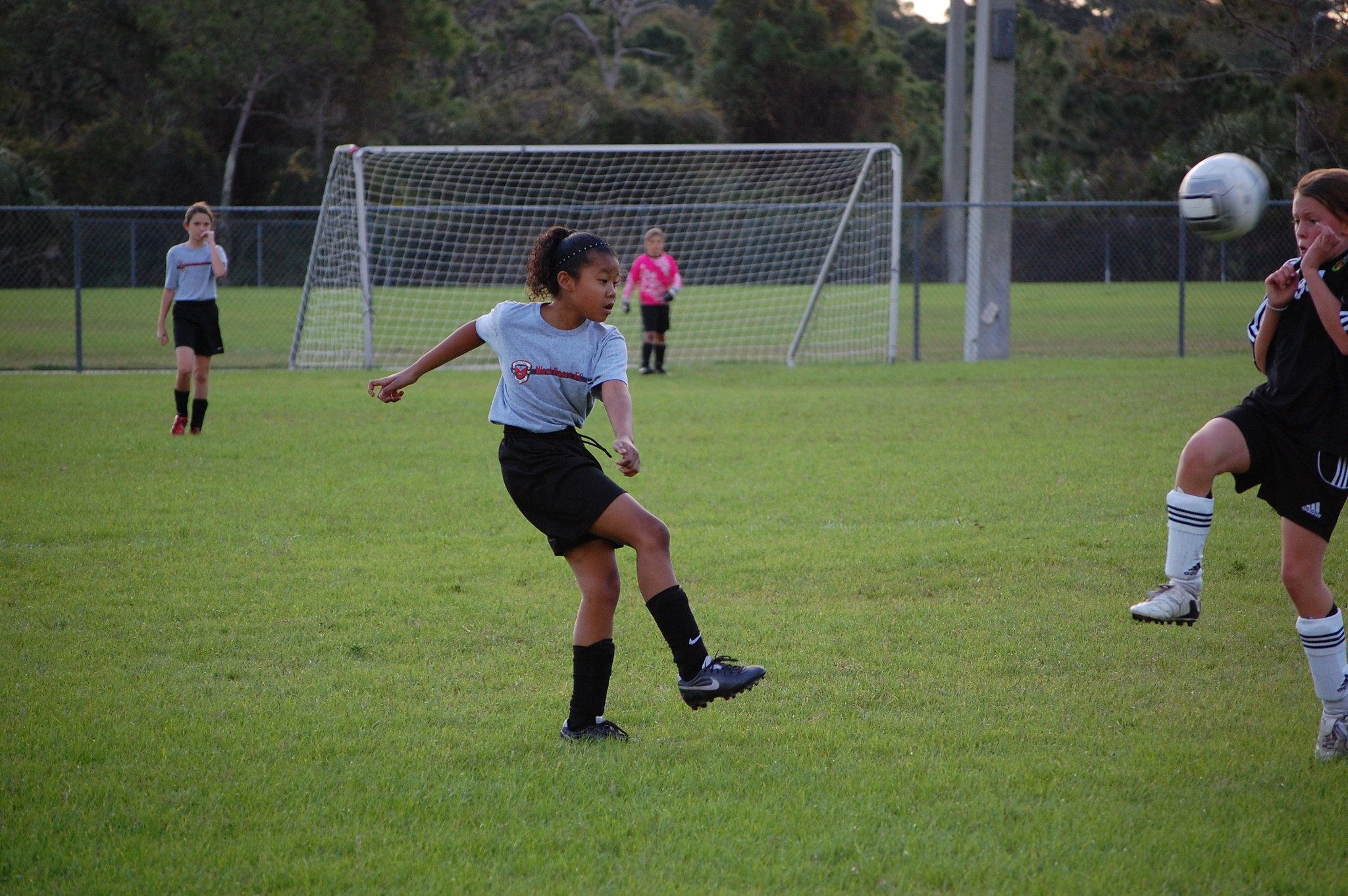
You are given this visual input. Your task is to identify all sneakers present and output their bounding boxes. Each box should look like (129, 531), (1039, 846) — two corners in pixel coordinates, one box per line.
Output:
(560, 715), (629, 742)
(1313, 710), (1348, 761)
(170, 415), (188, 435)
(676, 651), (766, 712)
(1131, 580), (1201, 629)
(191, 426), (201, 437)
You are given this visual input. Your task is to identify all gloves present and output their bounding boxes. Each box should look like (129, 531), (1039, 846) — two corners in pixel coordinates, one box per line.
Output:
(621, 302), (633, 316)
(664, 293), (673, 303)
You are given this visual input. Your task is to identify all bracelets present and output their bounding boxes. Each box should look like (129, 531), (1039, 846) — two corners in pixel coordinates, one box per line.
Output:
(1267, 302), (1289, 311)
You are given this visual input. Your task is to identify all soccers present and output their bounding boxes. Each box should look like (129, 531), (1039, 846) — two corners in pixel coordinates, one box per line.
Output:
(1180, 154), (1269, 240)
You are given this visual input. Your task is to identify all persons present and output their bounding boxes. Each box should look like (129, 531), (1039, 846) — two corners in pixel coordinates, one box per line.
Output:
(157, 201), (228, 436)
(1129, 169), (1348, 762)
(367, 225), (767, 747)
(621, 228), (682, 374)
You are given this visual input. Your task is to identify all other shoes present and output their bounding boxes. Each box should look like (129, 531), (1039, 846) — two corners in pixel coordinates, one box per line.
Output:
(655, 367), (666, 374)
(639, 367), (650, 375)
